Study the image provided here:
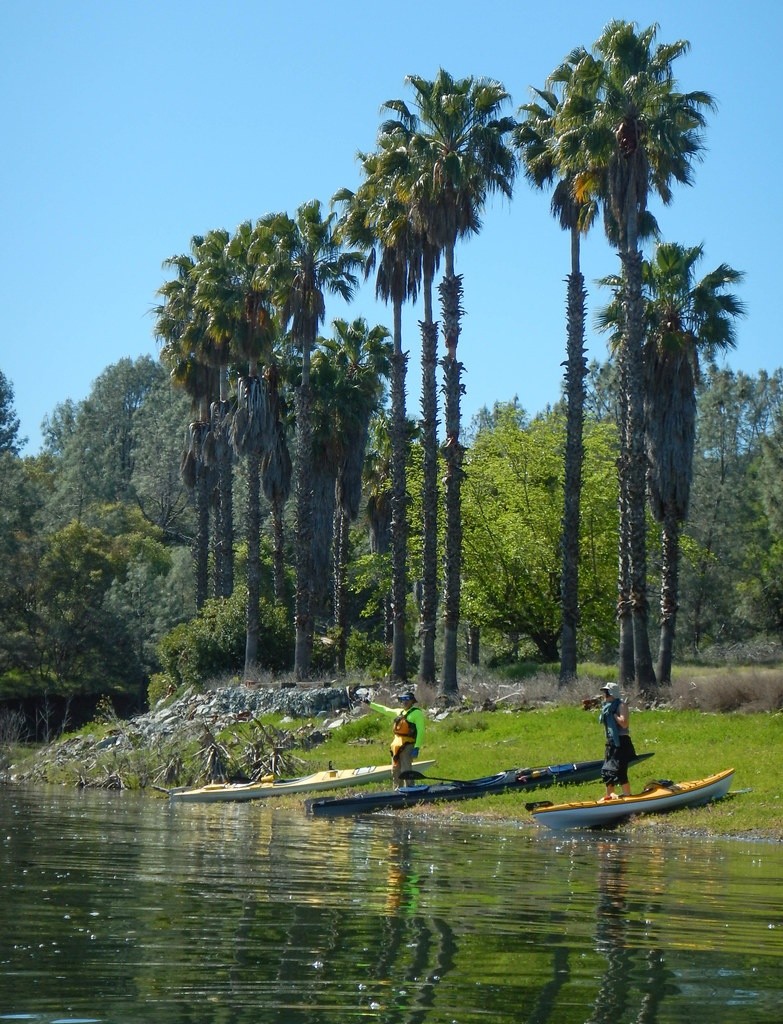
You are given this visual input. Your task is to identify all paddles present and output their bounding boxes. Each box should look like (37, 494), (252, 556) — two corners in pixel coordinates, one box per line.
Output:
(396, 770), (462, 783)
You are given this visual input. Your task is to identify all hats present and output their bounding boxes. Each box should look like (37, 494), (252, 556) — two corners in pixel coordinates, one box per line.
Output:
(398, 692), (418, 704)
(600, 682), (621, 699)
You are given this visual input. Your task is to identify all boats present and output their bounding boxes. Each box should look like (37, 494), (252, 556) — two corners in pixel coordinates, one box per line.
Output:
(304, 752), (655, 819)
(170, 759), (437, 802)
(528, 767), (737, 831)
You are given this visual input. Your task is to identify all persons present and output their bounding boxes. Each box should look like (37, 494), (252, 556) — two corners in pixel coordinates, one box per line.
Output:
(597, 682), (637, 801)
(362, 690), (425, 789)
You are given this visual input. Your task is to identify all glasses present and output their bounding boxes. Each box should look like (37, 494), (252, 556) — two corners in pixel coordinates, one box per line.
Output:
(400, 697), (410, 701)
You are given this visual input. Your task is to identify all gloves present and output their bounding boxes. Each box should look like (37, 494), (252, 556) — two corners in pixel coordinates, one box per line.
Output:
(410, 748), (419, 759)
